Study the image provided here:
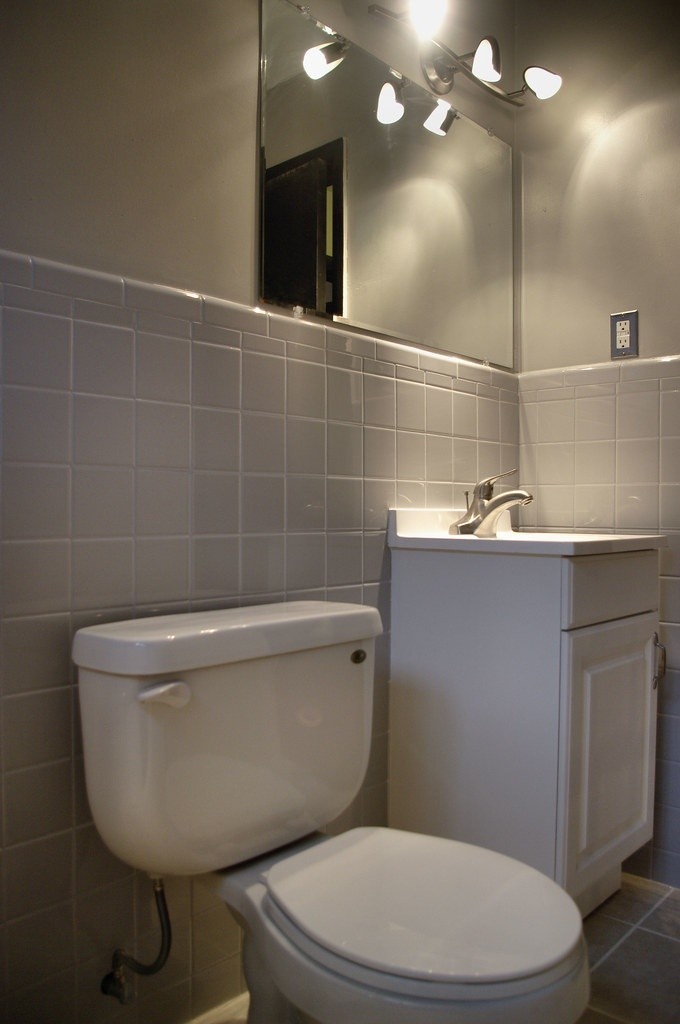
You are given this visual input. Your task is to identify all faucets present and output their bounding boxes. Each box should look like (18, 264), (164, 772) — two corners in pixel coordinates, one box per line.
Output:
(457, 467), (536, 537)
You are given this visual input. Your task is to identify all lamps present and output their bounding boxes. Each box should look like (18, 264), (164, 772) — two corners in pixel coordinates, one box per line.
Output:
(376, 78), (413, 124)
(421, 35), (502, 95)
(508, 66), (563, 100)
(422, 99), (455, 137)
(303, 42), (351, 80)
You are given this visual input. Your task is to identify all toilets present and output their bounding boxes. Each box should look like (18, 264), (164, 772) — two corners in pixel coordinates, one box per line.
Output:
(67, 596), (590, 1024)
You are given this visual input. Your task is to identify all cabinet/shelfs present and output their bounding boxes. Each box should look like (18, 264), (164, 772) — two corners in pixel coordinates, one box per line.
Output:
(390, 548), (667, 917)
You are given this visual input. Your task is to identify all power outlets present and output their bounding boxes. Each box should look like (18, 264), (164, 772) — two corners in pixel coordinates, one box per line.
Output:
(610, 310), (639, 361)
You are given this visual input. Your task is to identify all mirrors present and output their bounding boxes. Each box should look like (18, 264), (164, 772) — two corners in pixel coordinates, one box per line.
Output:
(258, 0), (514, 369)
